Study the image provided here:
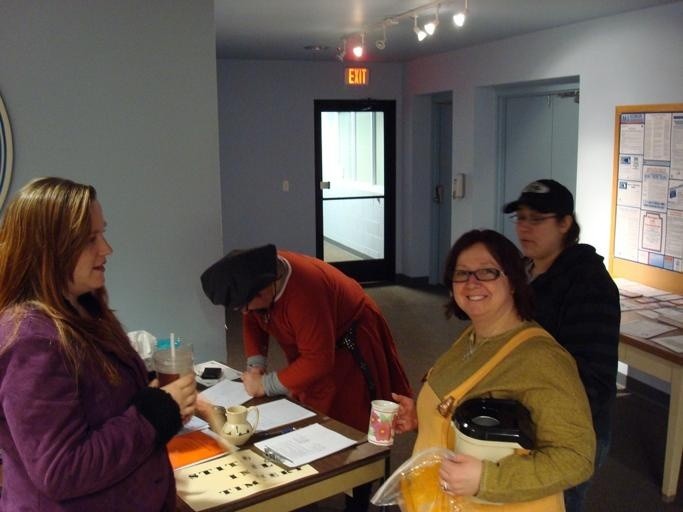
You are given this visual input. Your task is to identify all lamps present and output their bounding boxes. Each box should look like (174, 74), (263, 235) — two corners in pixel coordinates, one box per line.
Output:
(336, 0), (468, 63)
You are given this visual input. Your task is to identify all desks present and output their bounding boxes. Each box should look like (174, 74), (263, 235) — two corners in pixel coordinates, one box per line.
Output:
(614, 273), (681, 504)
(165, 356), (391, 512)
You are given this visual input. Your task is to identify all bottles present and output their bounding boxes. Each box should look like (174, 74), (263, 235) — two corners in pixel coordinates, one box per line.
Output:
(218, 403), (258, 448)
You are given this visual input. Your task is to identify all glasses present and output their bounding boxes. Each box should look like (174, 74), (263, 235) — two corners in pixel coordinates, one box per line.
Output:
(508, 214), (559, 226)
(446, 267), (500, 283)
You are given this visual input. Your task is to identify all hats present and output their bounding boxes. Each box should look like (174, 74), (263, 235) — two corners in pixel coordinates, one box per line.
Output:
(200, 244), (278, 313)
(501, 179), (573, 215)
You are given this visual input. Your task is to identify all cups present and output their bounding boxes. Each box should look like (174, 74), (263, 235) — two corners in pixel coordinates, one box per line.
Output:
(364, 400), (399, 446)
(152, 347), (195, 424)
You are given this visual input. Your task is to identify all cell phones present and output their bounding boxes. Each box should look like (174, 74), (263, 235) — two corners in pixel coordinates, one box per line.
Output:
(201, 368), (221, 379)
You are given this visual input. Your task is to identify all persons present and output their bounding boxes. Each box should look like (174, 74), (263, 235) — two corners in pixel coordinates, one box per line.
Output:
(391, 228), (598, 512)
(199, 243), (416, 512)
(500, 179), (621, 512)
(0, 175), (197, 511)
